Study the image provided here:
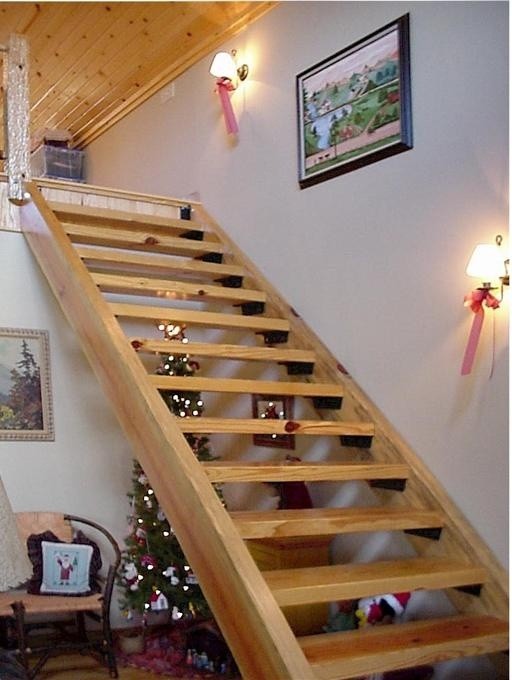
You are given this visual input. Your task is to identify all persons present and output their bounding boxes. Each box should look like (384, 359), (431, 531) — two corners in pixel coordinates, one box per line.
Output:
(55, 553), (74, 585)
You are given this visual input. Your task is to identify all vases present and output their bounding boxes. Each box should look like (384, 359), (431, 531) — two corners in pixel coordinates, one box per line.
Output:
(120, 630), (145, 657)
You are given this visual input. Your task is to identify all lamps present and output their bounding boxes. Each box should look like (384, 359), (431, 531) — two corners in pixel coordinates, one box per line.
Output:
(209, 52), (248, 81)
(464, 235), (509, 308)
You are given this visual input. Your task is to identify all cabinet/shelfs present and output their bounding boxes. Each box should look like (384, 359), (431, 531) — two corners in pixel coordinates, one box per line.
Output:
(241, 533), (336, 637)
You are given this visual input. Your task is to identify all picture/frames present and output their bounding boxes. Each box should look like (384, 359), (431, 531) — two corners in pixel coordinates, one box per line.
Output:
(296, 12), (413, 190)
(251, 393), (294, 449)
(0, 326), (55, 441)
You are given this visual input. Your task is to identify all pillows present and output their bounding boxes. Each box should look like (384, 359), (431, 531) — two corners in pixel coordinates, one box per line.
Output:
(27, 529), (103, 596)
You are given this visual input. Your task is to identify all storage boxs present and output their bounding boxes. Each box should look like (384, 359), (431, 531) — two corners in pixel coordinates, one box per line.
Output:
(43, 175), (85, 183)
(26, 145), (85, 179)
(28, 126), (73, 154)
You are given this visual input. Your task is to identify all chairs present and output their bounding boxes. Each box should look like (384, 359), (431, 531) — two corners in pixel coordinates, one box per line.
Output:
(0, 512), (121, 680)
